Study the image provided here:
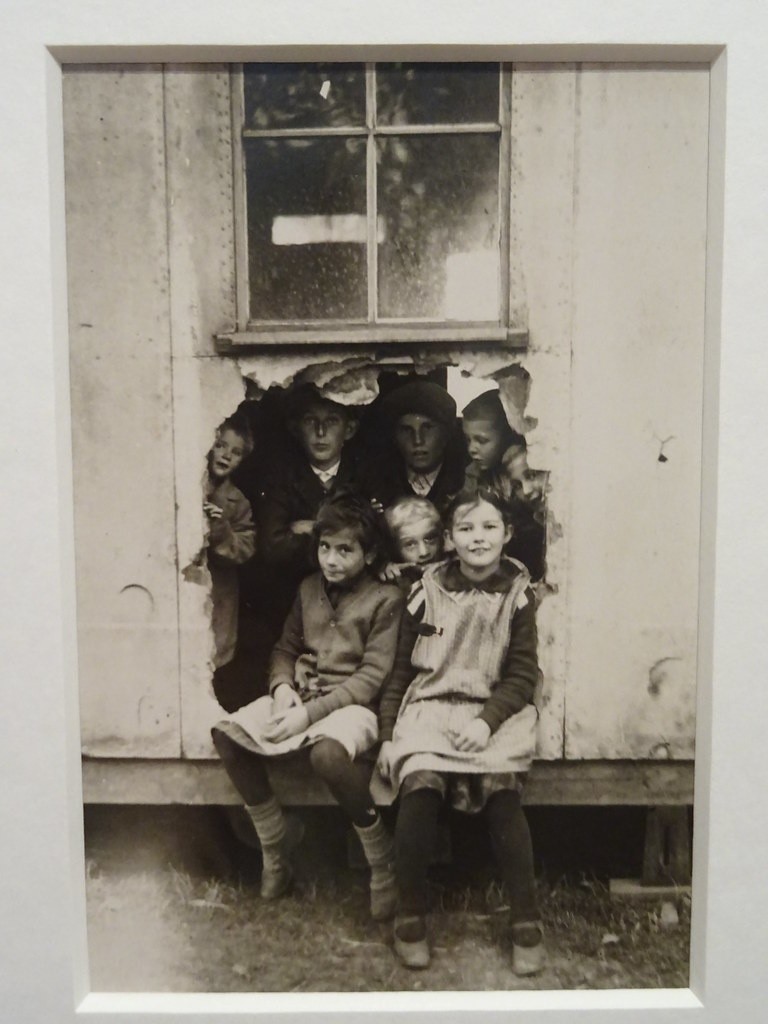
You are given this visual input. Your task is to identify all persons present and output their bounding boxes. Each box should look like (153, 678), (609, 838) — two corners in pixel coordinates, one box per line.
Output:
(377, 485), (558, 976)
(209, 494), (401, 920)
(205, 379), (541, 670)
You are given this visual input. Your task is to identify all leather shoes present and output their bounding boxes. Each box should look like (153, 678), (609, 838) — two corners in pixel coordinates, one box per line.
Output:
(393, 912), (430, 967)
(507, 912), (546, 976)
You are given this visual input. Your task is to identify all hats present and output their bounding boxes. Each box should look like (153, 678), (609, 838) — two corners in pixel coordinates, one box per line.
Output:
(377, 382), (457, 445)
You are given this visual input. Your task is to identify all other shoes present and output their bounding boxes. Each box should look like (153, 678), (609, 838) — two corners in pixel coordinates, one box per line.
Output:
(369, 860), (404, 921)
(260, 821), (304, 900)
